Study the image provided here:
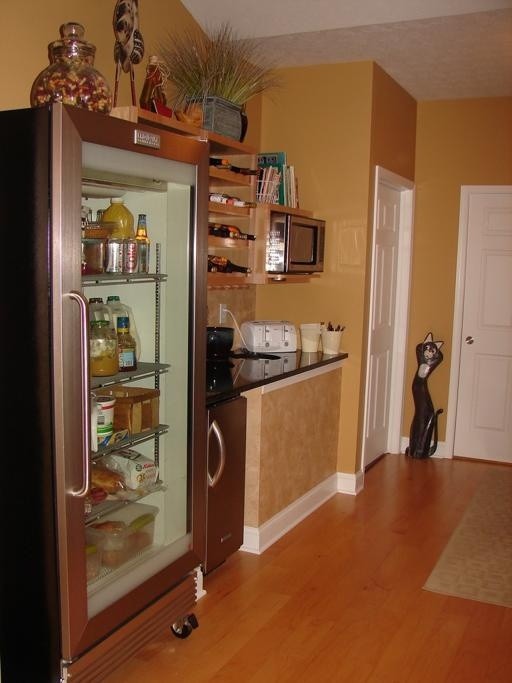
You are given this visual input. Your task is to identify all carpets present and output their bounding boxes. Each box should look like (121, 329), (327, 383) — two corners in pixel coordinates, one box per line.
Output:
(423, 468), (512, 610)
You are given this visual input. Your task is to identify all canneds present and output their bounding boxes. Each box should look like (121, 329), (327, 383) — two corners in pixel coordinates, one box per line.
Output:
(106, 238), (124, 272)
(89, 320), (119, 378)
(124, 237), (139, 274)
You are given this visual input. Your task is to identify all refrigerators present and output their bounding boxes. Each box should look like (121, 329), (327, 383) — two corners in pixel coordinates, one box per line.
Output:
(0, 103), (210, 681)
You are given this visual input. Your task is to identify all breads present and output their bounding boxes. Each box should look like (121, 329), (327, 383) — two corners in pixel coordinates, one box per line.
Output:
(91, 466), (124, 495)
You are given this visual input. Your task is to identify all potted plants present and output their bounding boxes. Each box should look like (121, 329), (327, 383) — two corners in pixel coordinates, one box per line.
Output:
(146, 17), (288, 142)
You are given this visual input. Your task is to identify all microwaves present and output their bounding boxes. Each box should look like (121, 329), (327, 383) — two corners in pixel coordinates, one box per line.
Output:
(270, 211), (328, 272)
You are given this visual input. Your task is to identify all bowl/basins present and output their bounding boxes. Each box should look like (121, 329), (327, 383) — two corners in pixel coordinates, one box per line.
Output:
(206, 368), (235, 392)
(207, 326), (234, 353)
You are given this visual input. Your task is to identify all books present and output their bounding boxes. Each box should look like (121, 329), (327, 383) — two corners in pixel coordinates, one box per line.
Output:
(256, 149), (300, 208)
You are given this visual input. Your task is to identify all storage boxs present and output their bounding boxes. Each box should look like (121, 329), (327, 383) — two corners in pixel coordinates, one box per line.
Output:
(92, 384), (160, 435)
(85, 526), (107, 582)
(87, 501), (160, 571)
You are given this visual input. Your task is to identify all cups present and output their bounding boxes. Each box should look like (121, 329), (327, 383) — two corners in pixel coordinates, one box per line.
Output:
(300, 323), (343, 354)
(299, 353), (339, 368)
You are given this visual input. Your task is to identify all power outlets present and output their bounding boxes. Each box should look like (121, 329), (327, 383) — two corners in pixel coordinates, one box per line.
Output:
(219, 304), (227, 324)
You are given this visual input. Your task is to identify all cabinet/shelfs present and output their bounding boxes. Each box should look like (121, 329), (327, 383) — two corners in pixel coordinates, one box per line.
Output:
(108, 106), (259, 288)
(207, 396), (247, 575)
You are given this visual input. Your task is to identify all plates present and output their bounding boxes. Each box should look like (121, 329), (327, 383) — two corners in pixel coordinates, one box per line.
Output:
(207, 351), (235, 359)
(206, 360), (235, 368)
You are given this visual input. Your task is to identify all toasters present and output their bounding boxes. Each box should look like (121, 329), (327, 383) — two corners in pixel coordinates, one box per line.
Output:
(240, 353), (298, 384)
(240, 321), (298, 352)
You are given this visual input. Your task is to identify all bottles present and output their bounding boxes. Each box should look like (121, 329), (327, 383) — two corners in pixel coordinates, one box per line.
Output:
(209, 255), (253, 275)
(208, 154), (261, 176)
(81, 195), (151, 276)
(210, 193), (256, 209)
(209, 221), (255, 241)
(139, 55), (172, 117)
